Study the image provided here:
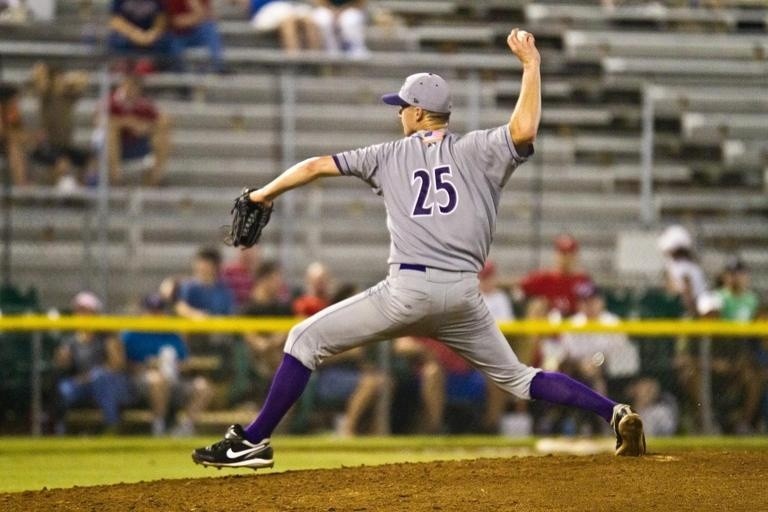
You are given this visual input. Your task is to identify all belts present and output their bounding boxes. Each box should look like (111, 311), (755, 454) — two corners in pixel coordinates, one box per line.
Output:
(395, 261), (431, 276)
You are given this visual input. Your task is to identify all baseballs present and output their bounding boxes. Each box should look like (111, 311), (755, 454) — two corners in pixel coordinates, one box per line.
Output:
(516, 30), (528, 41)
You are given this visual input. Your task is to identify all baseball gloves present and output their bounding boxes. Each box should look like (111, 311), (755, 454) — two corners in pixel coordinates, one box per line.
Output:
(219, 186), (274, 250)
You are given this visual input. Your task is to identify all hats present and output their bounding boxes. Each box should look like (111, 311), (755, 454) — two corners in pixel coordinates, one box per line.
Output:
(382, 72), (453, 114)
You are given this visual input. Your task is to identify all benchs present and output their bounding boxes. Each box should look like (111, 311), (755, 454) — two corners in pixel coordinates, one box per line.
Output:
(2, 1), (766, 425)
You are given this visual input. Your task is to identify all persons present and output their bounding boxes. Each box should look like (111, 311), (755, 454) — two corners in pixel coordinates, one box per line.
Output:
(184, 25), (651, 470)
(48, 247), (379, 438)
(248, 0), (370, 53)
(1, 64), (170, 188)
(659, 226), (767, 437)
(104, 1), (222, 75)
(0, 1), (58, 39)
(396, 236), (676, 440)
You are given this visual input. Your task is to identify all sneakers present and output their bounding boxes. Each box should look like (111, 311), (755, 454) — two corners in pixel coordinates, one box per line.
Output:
(613, 404), (646, 457)
(191, 423), (274, 471)
